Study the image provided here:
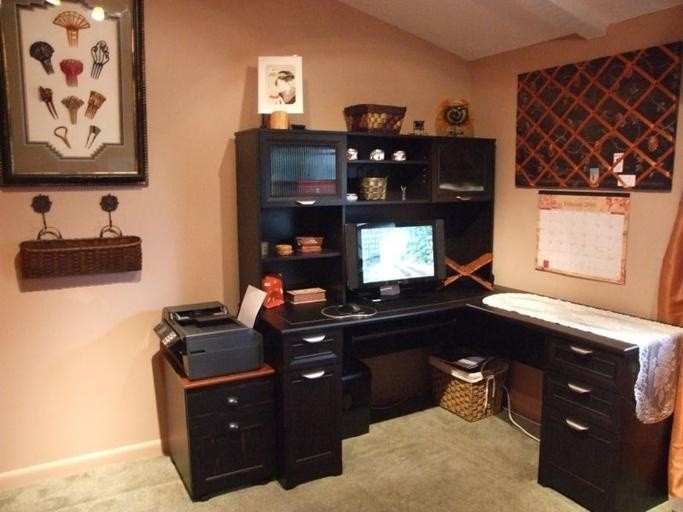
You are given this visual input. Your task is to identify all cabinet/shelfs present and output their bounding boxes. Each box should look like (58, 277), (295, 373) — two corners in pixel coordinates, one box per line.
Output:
(233, 126), (497, 304)
(159, 350), (279, 503)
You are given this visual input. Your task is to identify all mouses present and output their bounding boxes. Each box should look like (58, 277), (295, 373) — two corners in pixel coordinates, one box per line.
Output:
(341, 304), (361, 313)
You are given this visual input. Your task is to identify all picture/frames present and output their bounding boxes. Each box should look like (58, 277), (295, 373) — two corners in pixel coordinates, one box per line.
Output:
(0, 0), (150, 187)
(256, 55), (304, 116)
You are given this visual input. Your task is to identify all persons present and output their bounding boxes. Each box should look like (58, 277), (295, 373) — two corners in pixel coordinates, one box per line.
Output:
(268, 70), (295, 105)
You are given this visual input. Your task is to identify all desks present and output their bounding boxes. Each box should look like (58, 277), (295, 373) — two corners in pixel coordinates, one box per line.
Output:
(243, 283), (683, 512)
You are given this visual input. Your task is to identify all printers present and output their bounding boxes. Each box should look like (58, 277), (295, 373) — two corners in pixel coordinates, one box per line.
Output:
(152, 300), (264, 381)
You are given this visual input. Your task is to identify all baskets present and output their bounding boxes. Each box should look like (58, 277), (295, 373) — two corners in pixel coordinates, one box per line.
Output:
(429, 368), (507, 422)
(360, 178), (387, 200)
(343, 103), (407, 135)
(18, 235), (142, 277)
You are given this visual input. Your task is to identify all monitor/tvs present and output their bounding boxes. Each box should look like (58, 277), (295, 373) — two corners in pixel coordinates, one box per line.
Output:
(344, 219), (447, 304)
